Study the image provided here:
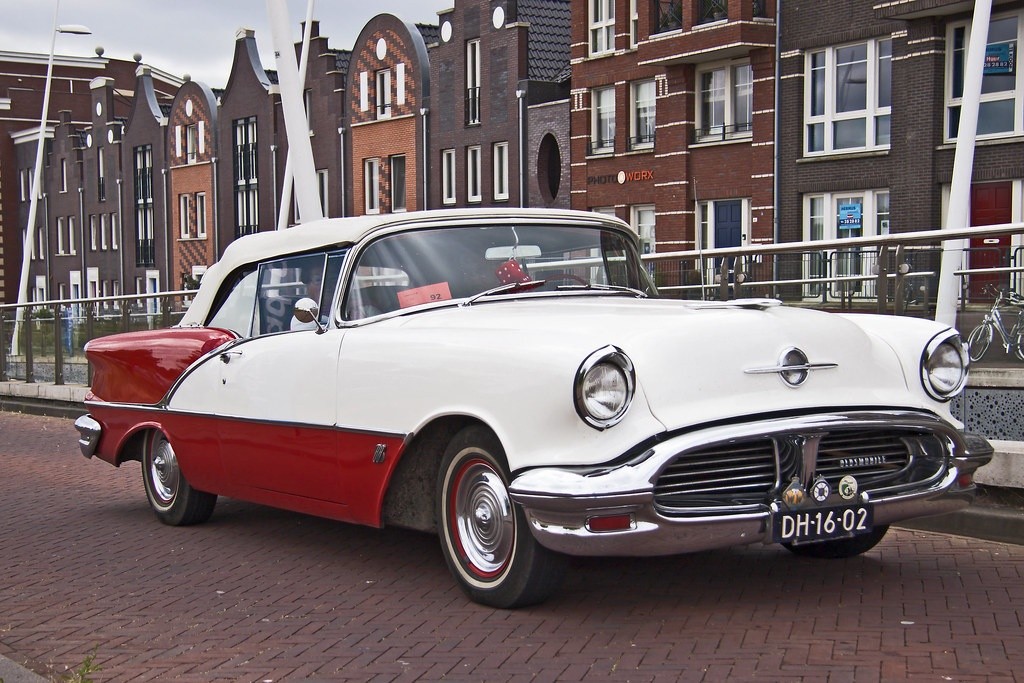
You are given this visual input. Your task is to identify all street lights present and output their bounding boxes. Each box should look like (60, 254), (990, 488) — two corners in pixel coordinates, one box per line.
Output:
(11, 0), (92, 355)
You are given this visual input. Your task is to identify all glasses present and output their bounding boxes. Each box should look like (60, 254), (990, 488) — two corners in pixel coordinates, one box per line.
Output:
(308, 276), (322, 285)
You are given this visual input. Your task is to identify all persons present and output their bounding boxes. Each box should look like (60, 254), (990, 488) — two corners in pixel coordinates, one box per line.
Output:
(290, 259), (381, 332)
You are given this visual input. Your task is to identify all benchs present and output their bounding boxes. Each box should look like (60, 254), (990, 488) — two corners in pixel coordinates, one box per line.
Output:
(260, 285), (413, 335)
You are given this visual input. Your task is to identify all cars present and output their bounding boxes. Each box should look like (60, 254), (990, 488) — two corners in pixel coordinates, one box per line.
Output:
(75, 208), (994, 608)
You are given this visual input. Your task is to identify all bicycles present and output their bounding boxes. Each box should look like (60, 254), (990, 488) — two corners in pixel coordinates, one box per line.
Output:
(966, 283), (1024, 363)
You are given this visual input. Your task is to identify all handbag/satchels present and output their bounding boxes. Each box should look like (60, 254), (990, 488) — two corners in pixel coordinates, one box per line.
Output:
(495, 260), (532, 292)
